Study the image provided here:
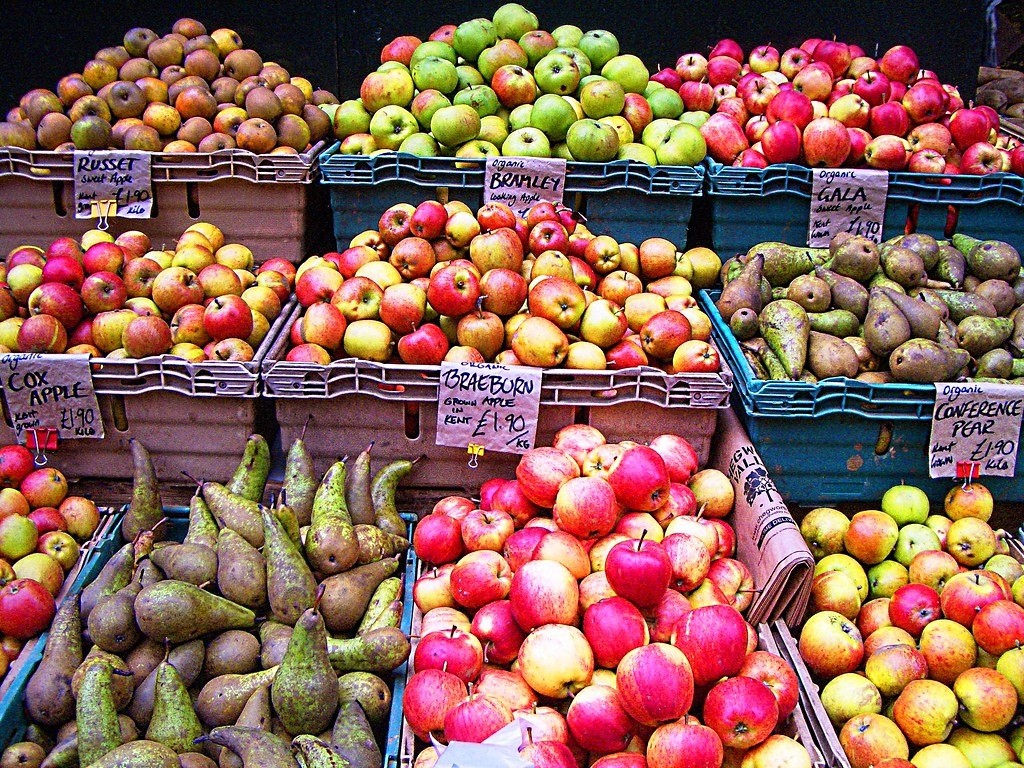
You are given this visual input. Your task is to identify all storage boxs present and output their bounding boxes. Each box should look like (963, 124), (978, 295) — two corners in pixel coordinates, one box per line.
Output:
(0, 110), (1024, 768)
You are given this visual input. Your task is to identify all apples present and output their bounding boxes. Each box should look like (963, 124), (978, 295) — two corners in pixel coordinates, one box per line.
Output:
(651, 34), (1024, 186)
(0, 17), (342, 158)
(795, 480), (1024, 768)
(335, 2), (712, 169)
(0, 221), (295, 674)
(400, 422), (813, 768)
(285, 199), (721, 375)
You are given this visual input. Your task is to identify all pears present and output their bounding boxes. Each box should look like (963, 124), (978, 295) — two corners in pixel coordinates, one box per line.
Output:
(718, 233), (1024, 388)
(0, 414), (420, 768)
